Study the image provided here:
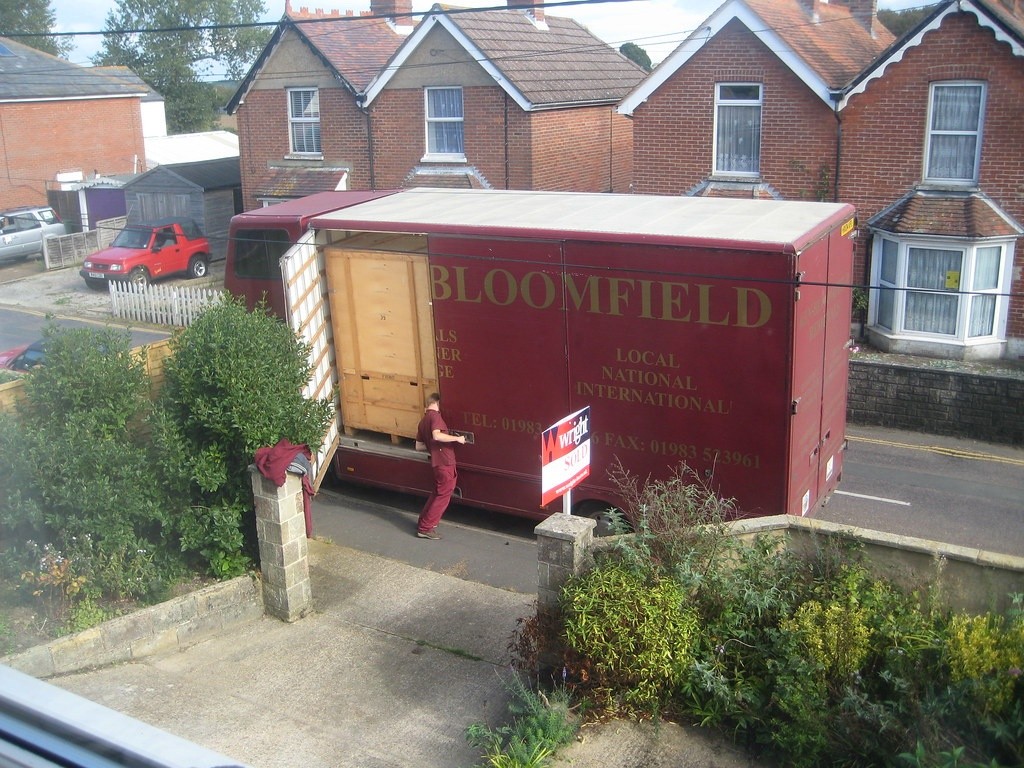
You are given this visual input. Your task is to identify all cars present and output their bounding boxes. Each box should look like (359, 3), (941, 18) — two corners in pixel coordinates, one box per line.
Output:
(0, 331), (109, 381)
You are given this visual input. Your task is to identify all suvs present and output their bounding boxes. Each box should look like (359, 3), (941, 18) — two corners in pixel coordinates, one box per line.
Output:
(0, 204), (67, 263)
(79, 216), (213, 293)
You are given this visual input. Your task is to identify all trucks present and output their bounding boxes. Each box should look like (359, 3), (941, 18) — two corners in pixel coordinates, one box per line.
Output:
(223, 185), (861, 538)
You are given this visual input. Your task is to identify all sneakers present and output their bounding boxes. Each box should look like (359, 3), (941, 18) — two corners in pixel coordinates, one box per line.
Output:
(418, 526), (442, 539)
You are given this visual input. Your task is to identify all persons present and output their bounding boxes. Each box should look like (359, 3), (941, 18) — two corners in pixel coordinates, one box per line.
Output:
(0, 217), (13, 229)
(416, 393), (467, 539)
